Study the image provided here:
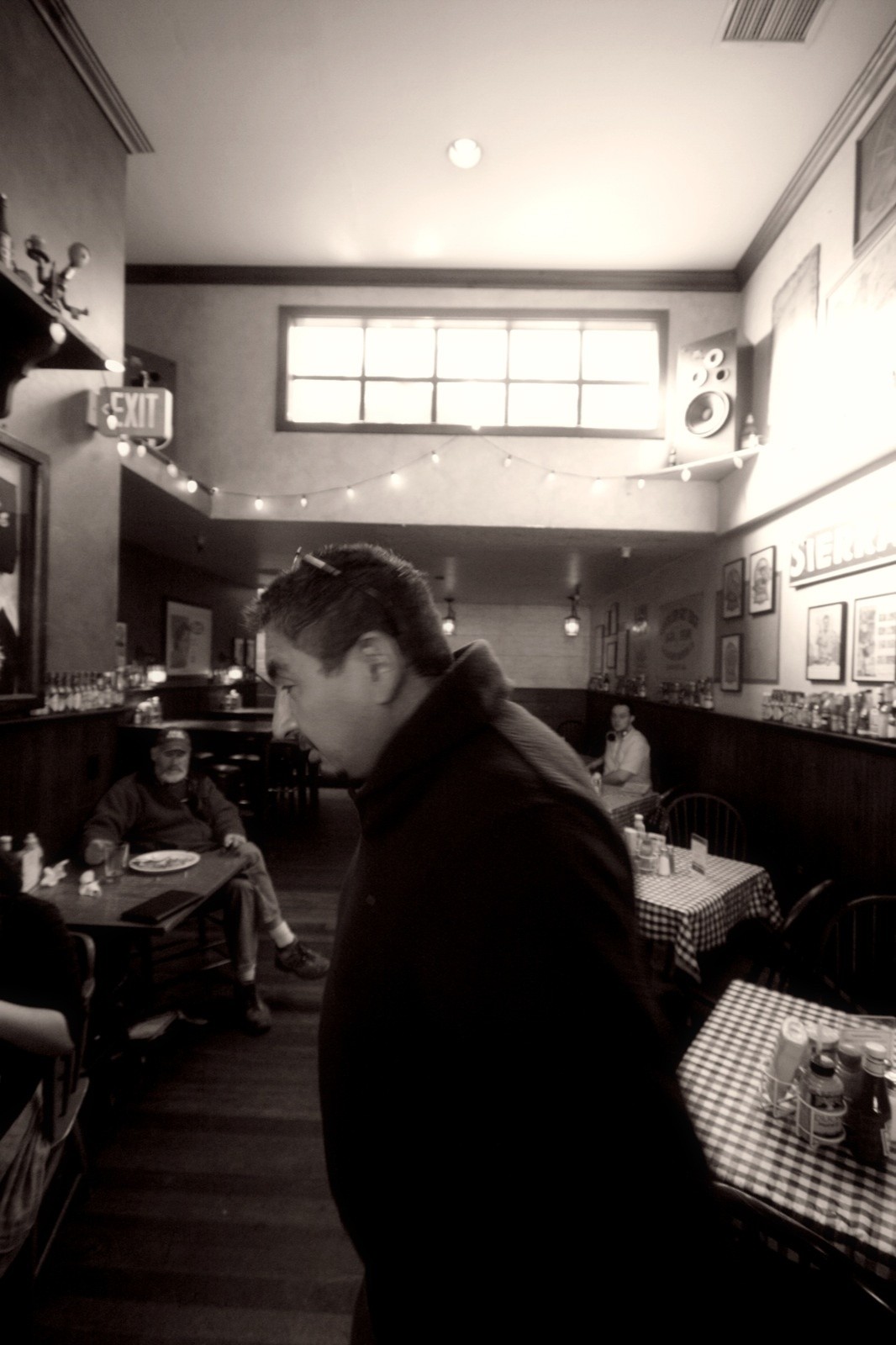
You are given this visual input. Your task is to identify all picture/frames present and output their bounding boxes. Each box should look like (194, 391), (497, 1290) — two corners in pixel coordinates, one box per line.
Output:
(851, 82), (896, 263)
(748, 545), (777, 615)
(851, 591), (896, 683)
(719, 632), (743, 693)
(805, 601), (847, 681)
(722, 557), (745, 620)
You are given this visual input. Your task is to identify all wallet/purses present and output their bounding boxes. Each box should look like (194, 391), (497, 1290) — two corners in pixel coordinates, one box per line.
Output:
(121, 889), (202, 924)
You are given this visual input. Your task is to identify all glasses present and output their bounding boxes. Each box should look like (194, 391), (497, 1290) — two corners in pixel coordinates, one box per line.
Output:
(292, 547), (393, 610)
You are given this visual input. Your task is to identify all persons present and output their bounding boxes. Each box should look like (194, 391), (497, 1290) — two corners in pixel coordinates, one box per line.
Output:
(242, 545), (734, 1345)
(587, 703), (650, 788)
(85, 727), (330, 1028)
(0, 841), (83, 1136)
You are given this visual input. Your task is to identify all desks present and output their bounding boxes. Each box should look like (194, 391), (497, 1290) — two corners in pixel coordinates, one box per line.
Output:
(137, 717), (275, 843)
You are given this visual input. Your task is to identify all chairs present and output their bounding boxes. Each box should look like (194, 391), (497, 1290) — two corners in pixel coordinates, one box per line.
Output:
(653, 794), (896, 1016)
(22, 928), (100, 1292)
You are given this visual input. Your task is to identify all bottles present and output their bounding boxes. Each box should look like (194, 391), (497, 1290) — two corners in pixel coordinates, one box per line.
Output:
(588, 671), (714, 710)
(41, 661), (244, 725)
(795, 1026), (896, 1167)
(0, 832), (44, 876)
(623, 813), (675, 876)
(761, 682), (896, 743)
(589, 768), (603, 797)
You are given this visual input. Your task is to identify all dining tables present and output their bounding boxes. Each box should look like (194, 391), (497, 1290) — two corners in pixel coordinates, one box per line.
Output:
(674, 977), (896, 1296)
(625, 842), (781, 983)
(27, 850), (251, 1044)
(592, 783), (658, 833)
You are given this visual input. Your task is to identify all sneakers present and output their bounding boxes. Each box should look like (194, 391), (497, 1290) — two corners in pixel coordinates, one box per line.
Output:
(236, 985), (272, 1029)
(275, 938), (331, 977)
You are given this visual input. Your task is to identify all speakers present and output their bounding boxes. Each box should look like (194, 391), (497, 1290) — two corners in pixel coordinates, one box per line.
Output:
(667, 328), (756, 468)
(122, 342), (177, 395)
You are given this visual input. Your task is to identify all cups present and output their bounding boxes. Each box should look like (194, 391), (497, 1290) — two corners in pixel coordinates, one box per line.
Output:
(103, 841), (130, 885)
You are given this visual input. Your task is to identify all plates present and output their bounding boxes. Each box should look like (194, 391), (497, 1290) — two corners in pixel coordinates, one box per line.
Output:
(129, 850), (200, 873)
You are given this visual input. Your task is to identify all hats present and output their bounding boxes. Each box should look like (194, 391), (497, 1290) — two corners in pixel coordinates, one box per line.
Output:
(157, 726), (191, 753)
